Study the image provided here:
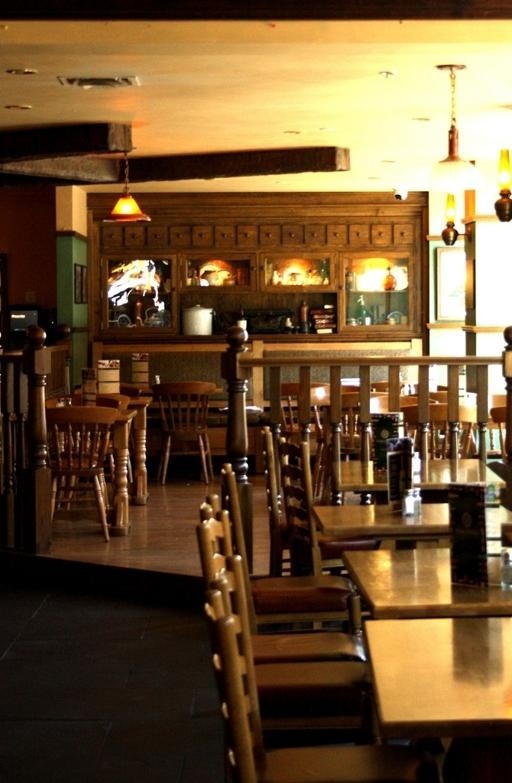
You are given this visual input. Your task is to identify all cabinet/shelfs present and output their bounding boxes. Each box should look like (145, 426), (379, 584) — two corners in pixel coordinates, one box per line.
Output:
(179, 249), (258, 293)
(257, 251), (339, 293)
(340, 251), (414, 333)
(100, 250), (176, 333)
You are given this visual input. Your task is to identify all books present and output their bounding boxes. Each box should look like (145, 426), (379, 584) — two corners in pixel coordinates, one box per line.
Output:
(385, 437), (414, 513)
(370, 412), (404, 471)
(448, 482), (501, 587)
(311, 304), (336, 334)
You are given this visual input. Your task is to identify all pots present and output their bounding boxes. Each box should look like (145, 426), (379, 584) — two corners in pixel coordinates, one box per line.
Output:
(181, 304), (216, 335)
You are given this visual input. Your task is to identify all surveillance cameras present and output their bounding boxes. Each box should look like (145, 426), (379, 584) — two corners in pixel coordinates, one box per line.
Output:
(394, 180), (408, 201)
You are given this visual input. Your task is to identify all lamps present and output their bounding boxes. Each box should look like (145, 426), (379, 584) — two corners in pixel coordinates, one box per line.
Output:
(429, 65), (477, 194)
(101, 125), (151, 224)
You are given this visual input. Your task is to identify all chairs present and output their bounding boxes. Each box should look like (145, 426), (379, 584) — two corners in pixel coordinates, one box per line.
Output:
(196, 510), (368, 783)
(153, 382), (217, 485)
(199, 493), (367, 660)
(217, 555), (419, 783)
(224, 465), (361, 632)
(264, 430), (376, 631)
(271, 376), (511, 489)
(48, 407), (119, 542)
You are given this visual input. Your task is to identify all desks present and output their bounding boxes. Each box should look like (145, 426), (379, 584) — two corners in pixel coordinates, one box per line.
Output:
(344, 546), (512, 619)
(364, 618), (512, 782)
(333, 459), (507, 491)
(313, 503), (512, 537)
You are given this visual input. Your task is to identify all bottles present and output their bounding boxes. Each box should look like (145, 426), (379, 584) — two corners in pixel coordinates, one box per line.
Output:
(191, 269), (201, 286)
(357, 295), (370, 325)
(320, 261), (329, 285)
(346, 258), (355, 290)
(56, 398), (65, 408)
(401, 487), (422, 516)
(383, 266), (398, 291)
(65, 397), (73, 407)
(271, 271), (279, 285)
(299, 299), (309, 334)
(498, 547), (512, 589)
